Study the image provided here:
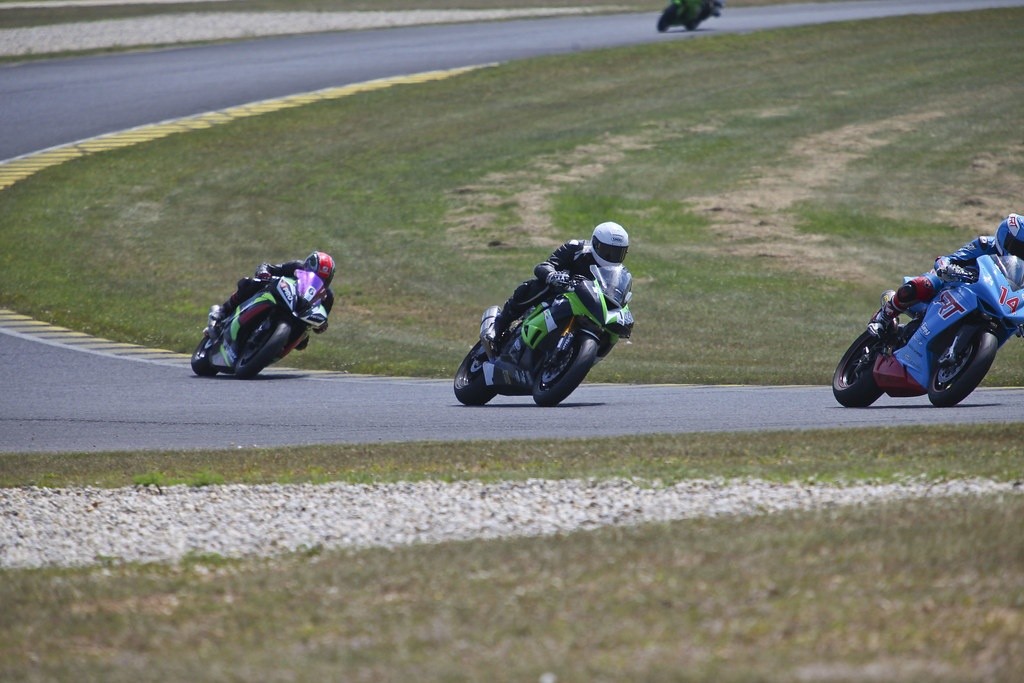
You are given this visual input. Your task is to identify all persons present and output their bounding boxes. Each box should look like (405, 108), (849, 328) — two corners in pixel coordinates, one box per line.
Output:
(485, 222), (635, 342)
(208, 251), (336, 352)
(709, 0), (724, 16)
(867, 214), (1024, 340)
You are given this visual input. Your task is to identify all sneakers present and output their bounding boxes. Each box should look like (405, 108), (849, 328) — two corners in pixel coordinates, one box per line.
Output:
(485, 313), (509, 343)
(210, 307), (226, 321)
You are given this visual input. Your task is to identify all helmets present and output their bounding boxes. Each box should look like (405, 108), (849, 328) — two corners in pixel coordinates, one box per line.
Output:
(995, 213), (1024, 256)
(591, 221), (629, 271)
(303, 251), (335, 287)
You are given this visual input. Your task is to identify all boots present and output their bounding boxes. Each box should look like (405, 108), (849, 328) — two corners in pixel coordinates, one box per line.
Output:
(868, 296), (906, 337)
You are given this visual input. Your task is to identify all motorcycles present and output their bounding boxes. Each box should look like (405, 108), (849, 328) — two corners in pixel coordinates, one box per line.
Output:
(830, 253), (1022, 406)
(190, 269), (329, 379)
(452, 263), (635, 408)
(656, 0), (722, 35)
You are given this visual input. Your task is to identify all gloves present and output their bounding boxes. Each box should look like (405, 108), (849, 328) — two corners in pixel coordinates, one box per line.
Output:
(939, 265), (967, 283)
(312, 321), (329, 334)
(545, 271), (571, 289)
(258, 271), (271, 280)
